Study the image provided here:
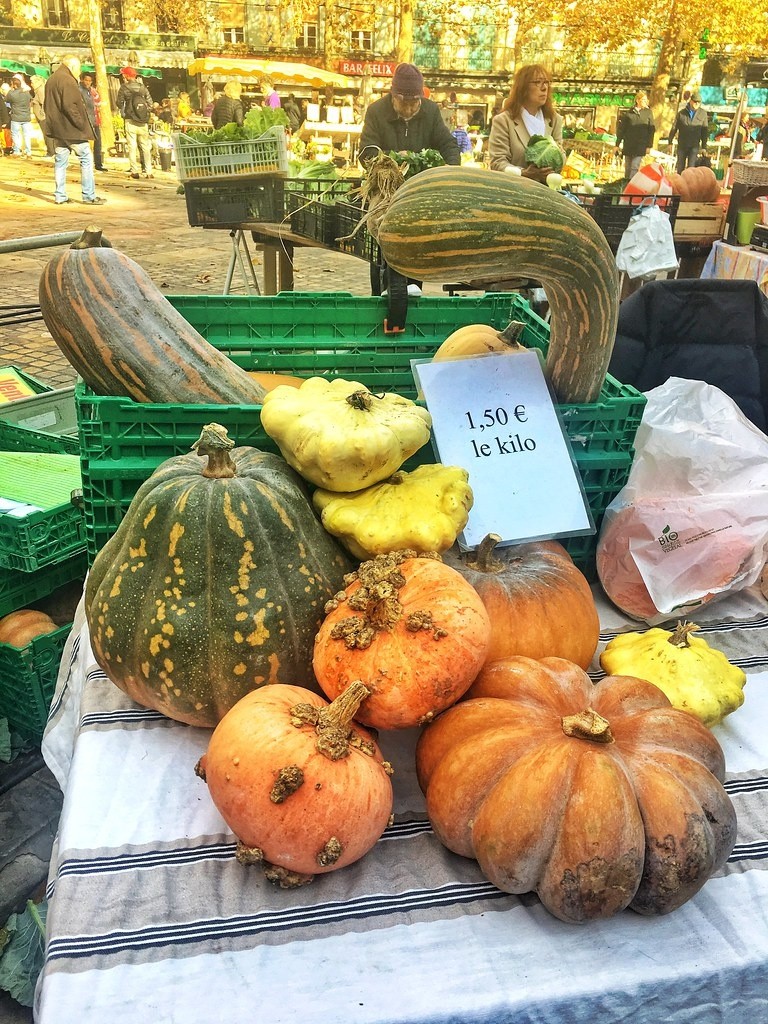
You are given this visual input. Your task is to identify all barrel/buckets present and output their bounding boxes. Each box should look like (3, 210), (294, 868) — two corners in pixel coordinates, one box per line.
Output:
(736, 207), (762, 245)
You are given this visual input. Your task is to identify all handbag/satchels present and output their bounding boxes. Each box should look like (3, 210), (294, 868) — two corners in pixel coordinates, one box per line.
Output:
(695, 153), (712, 169)
(597, 376), (768, 628)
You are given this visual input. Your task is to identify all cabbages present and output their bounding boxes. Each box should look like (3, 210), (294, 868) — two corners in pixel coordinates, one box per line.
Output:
(526, 134), (565, 173)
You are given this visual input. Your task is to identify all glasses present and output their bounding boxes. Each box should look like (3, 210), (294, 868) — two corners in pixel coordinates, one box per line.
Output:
(528, 80), (549, 87)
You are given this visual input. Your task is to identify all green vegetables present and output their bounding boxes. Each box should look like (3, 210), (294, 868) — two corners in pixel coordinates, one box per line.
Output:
(176, 105), (351, 218)
(389, 147), (446, 180)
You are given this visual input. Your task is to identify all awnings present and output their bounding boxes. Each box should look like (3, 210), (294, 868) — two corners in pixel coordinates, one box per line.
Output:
(187, 57), (348, 90)
(0, 58), (163, 80)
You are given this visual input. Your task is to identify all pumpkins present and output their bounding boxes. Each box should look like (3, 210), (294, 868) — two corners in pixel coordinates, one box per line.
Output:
(1, 162), (749, 923)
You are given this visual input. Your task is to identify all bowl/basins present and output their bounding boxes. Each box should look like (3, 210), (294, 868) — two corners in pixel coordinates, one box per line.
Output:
(618, 162), (672, 212)
(756, 195), (768, 225)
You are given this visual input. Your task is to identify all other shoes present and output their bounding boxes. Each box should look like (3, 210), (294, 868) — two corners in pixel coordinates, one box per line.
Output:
(141, 170), (146, 173)
(145, 174), (154, 179)
(130, 173), (140, 179)
(407, 284), (422, 297)
(83, 196), (107, 205)
(55, 198), (74, 204)
(97, 167), (108, 172)
(381, 288), (388, 296)
(0, 146), (32, 160)
(125, 169), (132, 172)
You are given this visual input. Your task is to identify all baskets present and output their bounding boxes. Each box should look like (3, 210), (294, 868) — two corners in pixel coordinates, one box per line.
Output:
(174, 125), (388, 259)
(733, 160), (767, 187)
(558, 184), (680, 245)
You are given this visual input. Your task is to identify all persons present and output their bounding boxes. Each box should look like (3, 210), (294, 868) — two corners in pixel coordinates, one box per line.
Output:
(116, 67), (154, 179)
(439, 99), (454, 132)
(258, 76), (281, 110)
(360, 63), (462, 296)
(153, 92), (218, 123)
(211, 81), (243, 129)
(45, 54), (108, 204)
(0, 73), (55, 159)
(615, 90), (655, 178)
(284, 93), (300, 134)
(727, 113), (768, 190)
(668, 93), (708, 175)
(489, 66), (567, 180)
(79, 72), (108, 171)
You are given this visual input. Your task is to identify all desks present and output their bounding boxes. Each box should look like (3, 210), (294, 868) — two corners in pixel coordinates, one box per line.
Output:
(699, 239), (768, 298)
(33, 568), (768, 1024)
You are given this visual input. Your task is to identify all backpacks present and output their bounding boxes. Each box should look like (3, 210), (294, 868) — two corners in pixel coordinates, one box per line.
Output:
(124, 83), (150, 123)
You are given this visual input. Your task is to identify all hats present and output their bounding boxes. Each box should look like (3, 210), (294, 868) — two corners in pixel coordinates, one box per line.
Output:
(120, 66), (136, 77)
(691, 93), (702, 102)
(391, 63), (425, 100)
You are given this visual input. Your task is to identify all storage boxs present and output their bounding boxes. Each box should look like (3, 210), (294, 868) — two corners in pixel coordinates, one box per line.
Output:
(0, 125), (726, 751)
